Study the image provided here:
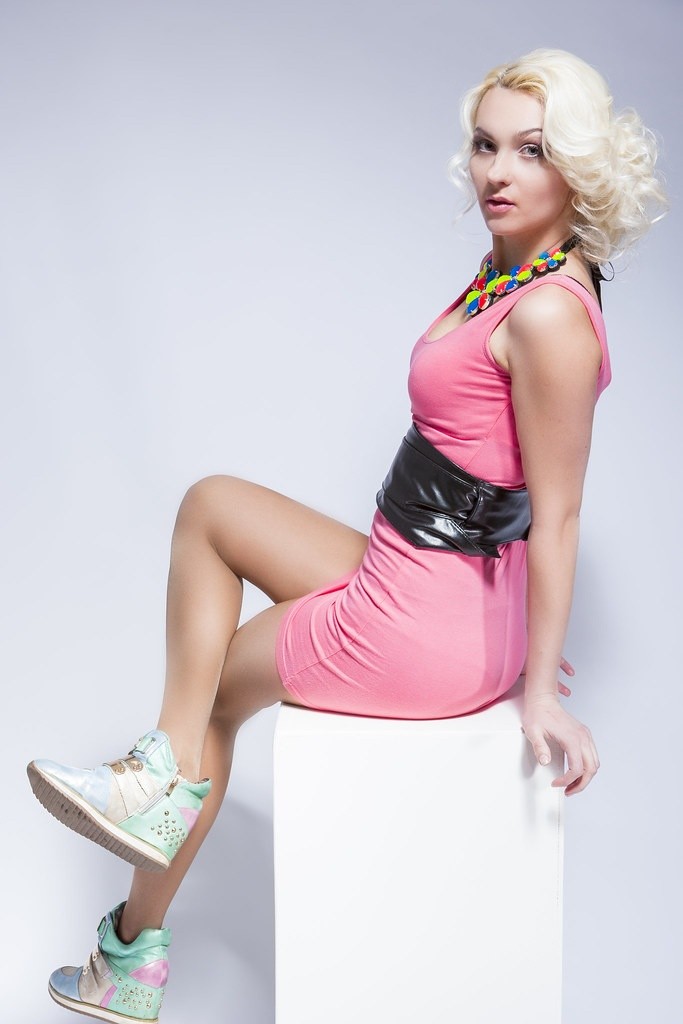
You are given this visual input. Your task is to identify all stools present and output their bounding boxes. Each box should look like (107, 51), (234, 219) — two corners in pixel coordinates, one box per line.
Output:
(275, 687), (565, 1024)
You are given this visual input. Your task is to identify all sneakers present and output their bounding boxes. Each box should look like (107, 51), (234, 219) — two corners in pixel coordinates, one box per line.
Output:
(48, 902), (172, 1024)
(27, 728), (212, 873)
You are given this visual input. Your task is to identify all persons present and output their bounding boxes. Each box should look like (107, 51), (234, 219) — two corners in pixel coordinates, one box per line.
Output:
(26, 50), (660, 1024)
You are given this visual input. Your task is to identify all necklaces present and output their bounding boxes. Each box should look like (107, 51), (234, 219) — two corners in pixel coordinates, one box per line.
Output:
(462, 235), (581, 317)
